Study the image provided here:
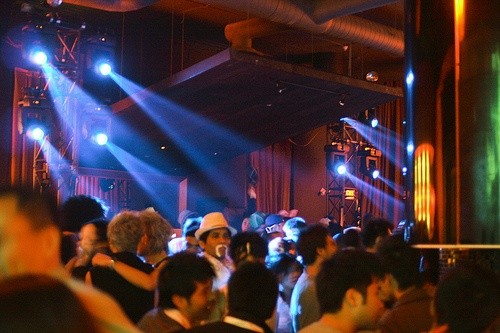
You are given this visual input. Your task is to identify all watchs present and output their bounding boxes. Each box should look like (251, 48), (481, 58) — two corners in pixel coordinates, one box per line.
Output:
(109, 258), (116, 269)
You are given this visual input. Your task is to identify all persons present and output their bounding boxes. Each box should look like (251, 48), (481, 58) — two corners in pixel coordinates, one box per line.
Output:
(0, 183), (500, 333)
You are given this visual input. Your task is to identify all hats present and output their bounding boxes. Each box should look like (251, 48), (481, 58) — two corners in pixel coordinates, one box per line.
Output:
(265, 214), (291, 226)
(178, 210), (199, 227)
(195, 212), (237, 240)
(228, 232), (268, 258)
